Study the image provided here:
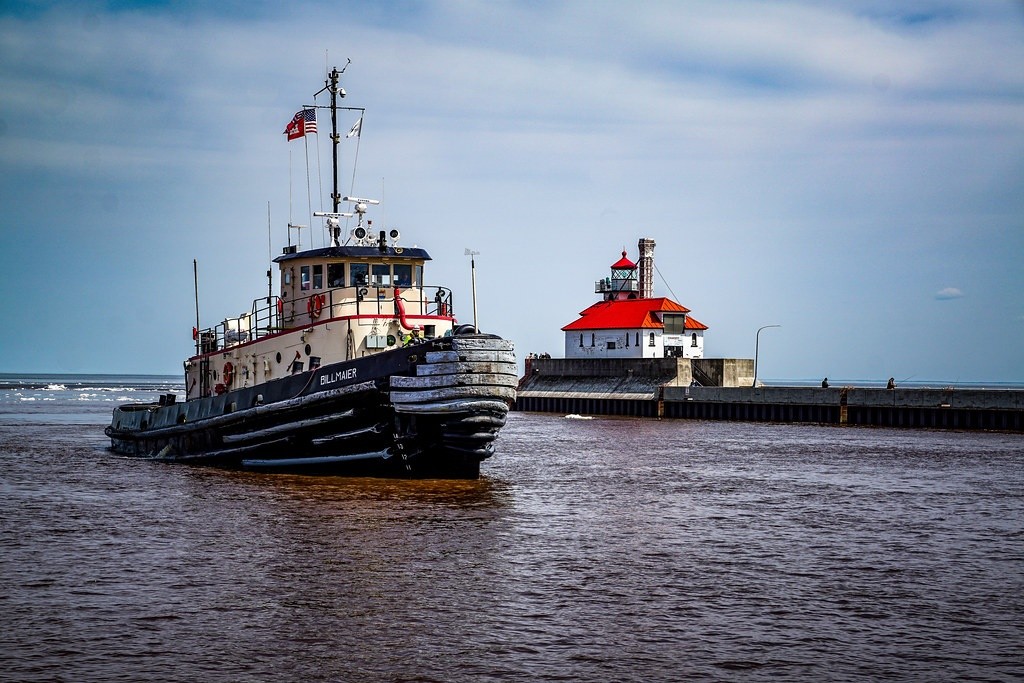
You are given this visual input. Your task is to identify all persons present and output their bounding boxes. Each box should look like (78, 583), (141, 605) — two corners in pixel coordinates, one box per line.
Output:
(529, 352), (551, 359)
(403, 326), (424, 347)
(887, 378), (897, 389)
(821, 378), (831, 388)
(395, 275), (410, 286)
(600, 277), (611, 292)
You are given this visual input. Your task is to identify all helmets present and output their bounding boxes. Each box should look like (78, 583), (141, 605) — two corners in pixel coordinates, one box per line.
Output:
(412, 325), (420, 331)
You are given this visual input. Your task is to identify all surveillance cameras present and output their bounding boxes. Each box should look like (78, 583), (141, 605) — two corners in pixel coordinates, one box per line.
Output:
(340, 88), (346, 98)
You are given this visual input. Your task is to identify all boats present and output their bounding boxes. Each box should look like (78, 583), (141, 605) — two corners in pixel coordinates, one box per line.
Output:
(104, 49), (519, 480)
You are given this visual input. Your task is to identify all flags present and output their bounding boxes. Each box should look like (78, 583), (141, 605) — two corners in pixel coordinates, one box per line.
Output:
(287, 117), (306, 141)
(284, 108), (317, 134)
(346, 117), (362, 138)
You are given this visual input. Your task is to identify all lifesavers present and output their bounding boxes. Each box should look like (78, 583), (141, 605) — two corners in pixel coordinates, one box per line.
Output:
(223, 362), (233, 385)
(308, 294), (322, 317)
